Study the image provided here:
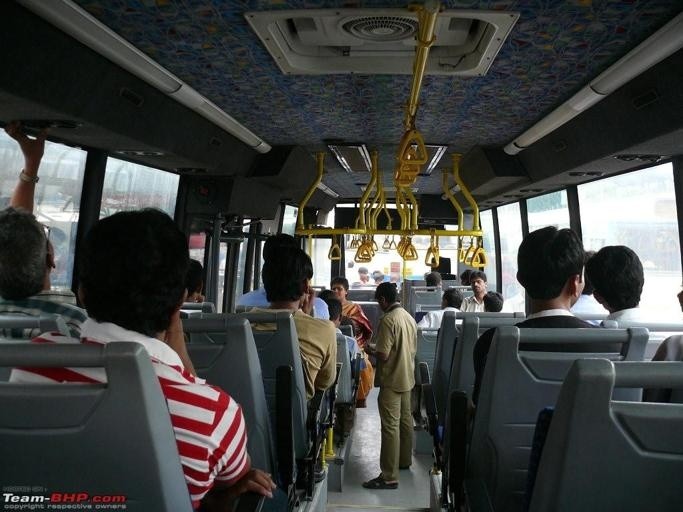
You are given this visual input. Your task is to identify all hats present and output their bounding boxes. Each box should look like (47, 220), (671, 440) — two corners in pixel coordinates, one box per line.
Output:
(375, 282), (397, 297)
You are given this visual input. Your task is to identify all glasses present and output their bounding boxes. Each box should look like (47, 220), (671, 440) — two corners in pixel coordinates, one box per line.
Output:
(42, 222), (51, 241)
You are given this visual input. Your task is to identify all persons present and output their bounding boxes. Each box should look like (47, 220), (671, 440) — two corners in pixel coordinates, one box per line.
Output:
(0, 206), (645, 512)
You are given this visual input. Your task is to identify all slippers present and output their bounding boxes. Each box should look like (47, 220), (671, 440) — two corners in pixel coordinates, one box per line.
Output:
(362, 476), (398, 489)
